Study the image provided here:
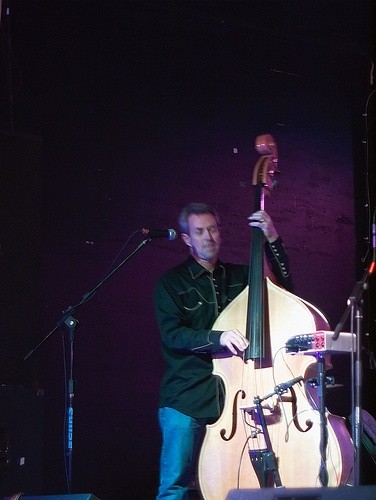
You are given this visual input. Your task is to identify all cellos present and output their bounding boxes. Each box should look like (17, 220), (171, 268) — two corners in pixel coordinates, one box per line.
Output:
(199, 133), (353, 500)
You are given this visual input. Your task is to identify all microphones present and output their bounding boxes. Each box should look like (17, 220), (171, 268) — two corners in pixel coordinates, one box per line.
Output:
(141, 228), (177, 240)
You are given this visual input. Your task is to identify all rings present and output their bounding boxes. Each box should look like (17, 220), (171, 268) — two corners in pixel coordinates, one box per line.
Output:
(260, 218), (266, 222)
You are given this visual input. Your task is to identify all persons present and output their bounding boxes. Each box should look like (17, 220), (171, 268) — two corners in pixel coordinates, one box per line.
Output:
(154, 201), (294, 500)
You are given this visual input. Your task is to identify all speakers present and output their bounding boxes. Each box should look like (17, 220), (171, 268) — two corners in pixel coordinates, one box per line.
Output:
(0, 385), (45, 496)
